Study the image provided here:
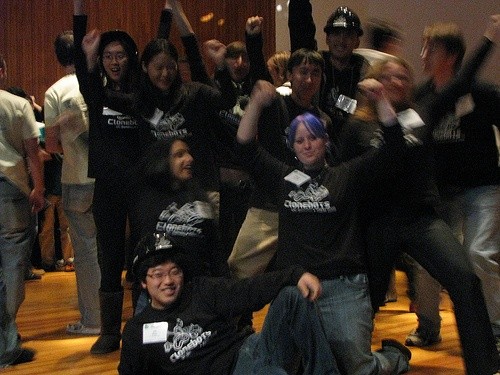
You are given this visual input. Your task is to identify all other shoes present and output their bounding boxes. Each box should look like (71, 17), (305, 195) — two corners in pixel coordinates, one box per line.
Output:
(10, 349), (33, 364)
(47, 268), (48, 268)
(41, 260), (56, 272)
(405, 332), (442, 348)
(28, 273), (41, 280)
(382, 339), (411, 361)
(410, 304), (417, 313)
(57, 258), (75, 271)
(67, 320), (101, 335)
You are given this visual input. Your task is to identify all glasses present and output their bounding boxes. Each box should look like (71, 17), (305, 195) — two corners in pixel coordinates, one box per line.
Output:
(100, 52), (127, 62)
(148, 266), (184, 280)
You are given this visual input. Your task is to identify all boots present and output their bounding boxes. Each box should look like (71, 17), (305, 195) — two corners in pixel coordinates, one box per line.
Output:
(90, 288), (125, 354)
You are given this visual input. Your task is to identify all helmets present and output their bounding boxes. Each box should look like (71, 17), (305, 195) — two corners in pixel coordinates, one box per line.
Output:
(323, 5), (364, 36)
(126, 231), (181, 283)
(96, 31), (138, 67)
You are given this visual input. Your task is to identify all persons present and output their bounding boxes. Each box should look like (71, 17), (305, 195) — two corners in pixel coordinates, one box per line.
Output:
(118, 233), (341, 375)
(343, 15), (500, 375)
(0, 61), (43, 368)
(236, 80), (411, 375)
(5, 0), (500, 355)
